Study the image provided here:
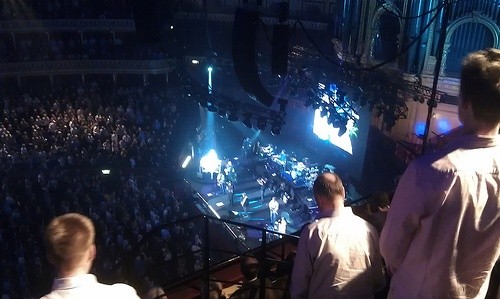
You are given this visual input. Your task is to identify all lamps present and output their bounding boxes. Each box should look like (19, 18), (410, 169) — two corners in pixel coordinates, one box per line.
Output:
(181, 80), (287, 136)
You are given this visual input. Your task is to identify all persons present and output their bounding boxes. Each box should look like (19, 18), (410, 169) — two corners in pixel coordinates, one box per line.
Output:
(378, 48), (500, 299)
(0, 80), (354, 299)
(289, 171), (389, 299)
(366, 188), (391, 235)
